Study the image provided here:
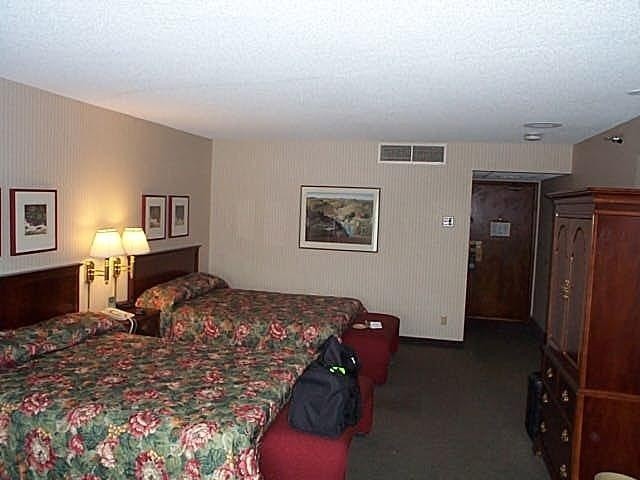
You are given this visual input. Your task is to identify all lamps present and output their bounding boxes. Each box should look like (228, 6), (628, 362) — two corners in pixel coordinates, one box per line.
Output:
(84, 227), (127, 287)
(112, 227), (152, 280)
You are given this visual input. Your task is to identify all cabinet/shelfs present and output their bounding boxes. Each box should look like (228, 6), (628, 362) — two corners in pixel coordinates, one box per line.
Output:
(530, 185), (640, 479)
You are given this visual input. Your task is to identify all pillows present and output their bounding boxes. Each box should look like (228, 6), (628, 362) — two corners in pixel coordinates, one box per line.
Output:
(0, 309), (127, 368)
(134, 271), (231, 306)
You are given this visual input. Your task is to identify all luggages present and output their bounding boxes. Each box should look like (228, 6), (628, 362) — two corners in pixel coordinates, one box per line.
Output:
(523, 370), (545, 442)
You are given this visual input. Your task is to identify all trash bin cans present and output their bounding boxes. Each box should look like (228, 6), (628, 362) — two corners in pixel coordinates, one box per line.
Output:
(593, 472), (637, 480)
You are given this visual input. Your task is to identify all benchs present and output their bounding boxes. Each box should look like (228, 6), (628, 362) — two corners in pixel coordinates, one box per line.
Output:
(343, 313), (400, 386)
(256, 374), (375, 479)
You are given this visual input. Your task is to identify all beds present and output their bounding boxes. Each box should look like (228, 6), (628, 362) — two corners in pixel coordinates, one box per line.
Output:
(127, 243), (366, 354)
(0, 262), (375, 479)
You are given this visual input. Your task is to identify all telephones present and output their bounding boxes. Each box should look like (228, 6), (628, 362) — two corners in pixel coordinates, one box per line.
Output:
(98, 307), (135, 321)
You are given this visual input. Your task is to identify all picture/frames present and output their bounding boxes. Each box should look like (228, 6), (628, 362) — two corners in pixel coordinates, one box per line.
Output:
(168, 194), (189, 238)
(142, 194), (167, 241)
(8, 187), (58, 256)
(296, 184), (381, 253)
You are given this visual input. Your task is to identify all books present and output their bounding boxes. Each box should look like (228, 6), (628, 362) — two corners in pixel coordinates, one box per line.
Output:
(370, 321), (382, 330)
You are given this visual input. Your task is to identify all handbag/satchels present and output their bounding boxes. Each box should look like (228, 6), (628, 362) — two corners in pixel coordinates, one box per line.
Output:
(287, 333), (363, 442)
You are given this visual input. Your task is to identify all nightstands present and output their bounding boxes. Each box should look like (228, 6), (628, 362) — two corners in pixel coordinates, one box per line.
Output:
(116, 306), (163, 338)
